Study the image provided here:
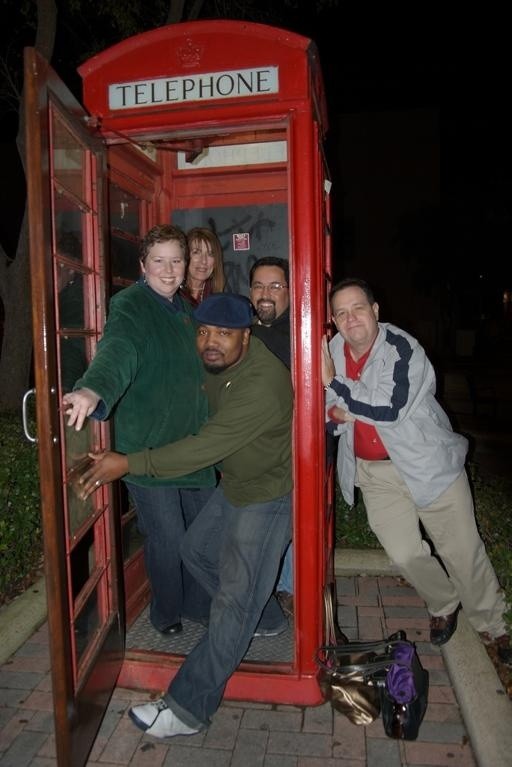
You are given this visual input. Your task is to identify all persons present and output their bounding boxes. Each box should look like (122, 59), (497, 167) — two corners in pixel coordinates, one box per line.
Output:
(78, 291), (298, 740)
(62, 222), (221, 638)
(318, 283), (511, 674)
(240, 258), (298, 594)
(172, 225), (225, 308)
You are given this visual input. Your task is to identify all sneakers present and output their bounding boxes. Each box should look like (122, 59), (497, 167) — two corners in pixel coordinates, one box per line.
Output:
(275, 590), (293, 619)
(128, 697), (201, 741)
(495, 634), (511, 666)
(430, 603), (461, 645)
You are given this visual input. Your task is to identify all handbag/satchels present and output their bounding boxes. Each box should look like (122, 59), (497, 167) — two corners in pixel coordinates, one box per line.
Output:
(383, 631), (428, 741)
(317, 642), (382, 725)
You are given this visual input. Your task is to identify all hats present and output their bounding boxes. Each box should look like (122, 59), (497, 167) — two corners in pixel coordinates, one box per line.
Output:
(194, 293), (252, 328)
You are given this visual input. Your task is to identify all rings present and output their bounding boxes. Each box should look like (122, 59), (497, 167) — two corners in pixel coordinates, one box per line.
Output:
(96, 480), (102, 487)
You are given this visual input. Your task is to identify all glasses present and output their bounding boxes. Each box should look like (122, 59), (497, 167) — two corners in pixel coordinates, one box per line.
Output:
(250, 283), (286, 293)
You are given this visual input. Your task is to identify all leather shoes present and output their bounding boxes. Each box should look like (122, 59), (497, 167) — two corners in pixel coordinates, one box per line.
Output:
(161, 621), (182, 637)
